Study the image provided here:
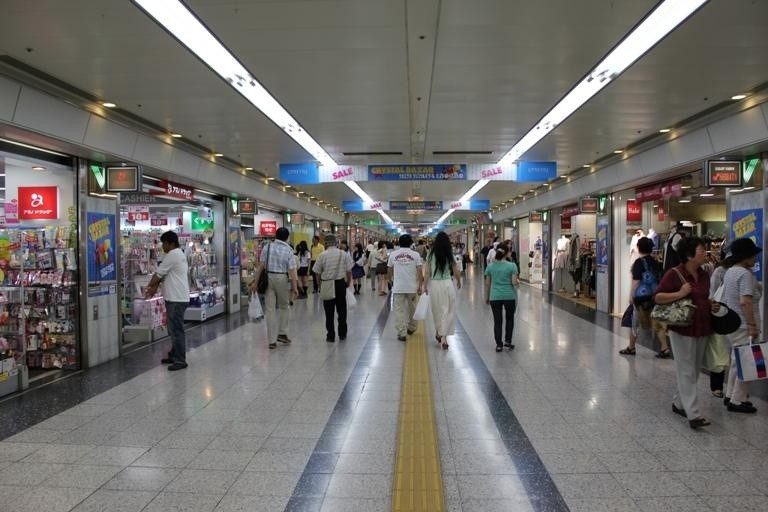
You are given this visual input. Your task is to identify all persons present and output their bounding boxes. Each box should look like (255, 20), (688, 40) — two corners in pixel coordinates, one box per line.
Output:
(722, 238), (759, 415)
(556, 234), (572, 292)
(296, 241), (310, 298)
(628, 229), (645, 288)
(481, 239), (492, 276)
(376, 242), (388, 296)
(367, 242), (379, 291)
(709, 248), (733, 398)
(646, 229), (659, 255)
(534, 236), (543, 251)
(387, 234), (424, 341)
(250, 227), (299, 349)
(313, 235), (354, 342)
(652, 237), (711, 430)
(665, 228), (682, 275)
(569, 233), (581, 297)
(484, 243), (520, 353)
(340, 240), (350, 256)
(144, 230), (190, 370)
(351, 243), (365, 295)
(288, 243), (301, 306)
(311, 236), (325, 294)
(420, 231), (462, 349)
(619, 237), (672, 359)
(415, 240), (428, 261)
(365, 240), (373, 256)
(486, 241), (498, 265)
(503, 239), (517, 262)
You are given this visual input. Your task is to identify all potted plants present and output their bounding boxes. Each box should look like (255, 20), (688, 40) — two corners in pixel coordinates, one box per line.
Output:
(245, 240), (256, 275)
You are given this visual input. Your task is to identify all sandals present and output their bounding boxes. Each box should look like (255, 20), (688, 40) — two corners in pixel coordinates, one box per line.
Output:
(619, 347), (636, 356)
(689, 417), (711, 430)
(655, 348), (672, 359)
(672, 404), (687, 419)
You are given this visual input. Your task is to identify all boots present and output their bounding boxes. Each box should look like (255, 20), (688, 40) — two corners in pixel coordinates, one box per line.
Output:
(302, 287), (308, 298)
(357, 285), (361, 296)
(353, 285), (358, 295)
(297, 287), (304, 299)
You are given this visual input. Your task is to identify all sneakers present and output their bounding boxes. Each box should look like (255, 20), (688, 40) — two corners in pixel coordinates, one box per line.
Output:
(379, 294), (384, 297)
(326, 338), (333, 343)
(269, 343), (277, 349)
(712, 391), (723, 399)
(312, 291), (317, 294)
(407, 329), (413, 336)
(497, 347), (502, 353)
(168, 363), (187, 372)
(503, 344), (515, 351)
(727, 402), (758, 414)
(339, 337), (345, 341)
(372, 289), (376, 291)
(381, 291), (387, 295)
(277, 335), (291, 343)
(724, 397), (752, 407)
(289, 301), (293, 307)
(161, 359), (173, 364)
(399, 337), (406, 342)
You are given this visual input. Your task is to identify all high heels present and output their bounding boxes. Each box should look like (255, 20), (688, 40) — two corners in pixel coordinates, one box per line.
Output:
(435, 336), (440, 345)
(442, 344), (449, 351)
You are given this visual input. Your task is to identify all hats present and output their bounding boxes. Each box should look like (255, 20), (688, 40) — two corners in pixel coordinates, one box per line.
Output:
(722, 239), (762, 263)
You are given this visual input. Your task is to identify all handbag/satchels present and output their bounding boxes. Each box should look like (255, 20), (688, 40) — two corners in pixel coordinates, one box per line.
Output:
(248, 289), (264, 320)
(634, 258), (658, 298)
(734, 336), (768, 383)
(620, 303), (634, 329)
(319, 251), (341, 300)
(257, 243), (270, 295)
(651, 269), (695, 327)
(701, 299), (730, 373)
(413, 293), (429, 322)
